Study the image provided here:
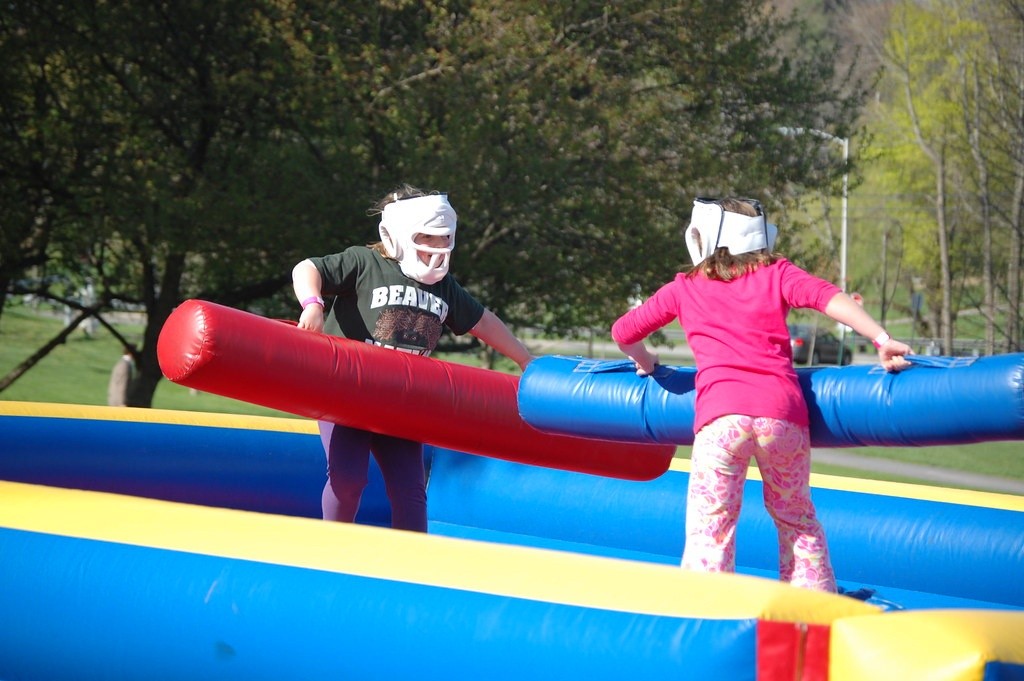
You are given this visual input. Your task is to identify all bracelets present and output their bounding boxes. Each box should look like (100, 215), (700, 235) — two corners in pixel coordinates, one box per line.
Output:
(301, 296), (325, 310)
(873, 331), (891, 348)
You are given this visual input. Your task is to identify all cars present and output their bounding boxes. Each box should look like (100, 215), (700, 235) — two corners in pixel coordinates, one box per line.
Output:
(783, 323), (853, 367)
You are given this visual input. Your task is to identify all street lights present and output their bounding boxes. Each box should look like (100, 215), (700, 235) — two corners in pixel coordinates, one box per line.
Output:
(777, 125), (849, 368)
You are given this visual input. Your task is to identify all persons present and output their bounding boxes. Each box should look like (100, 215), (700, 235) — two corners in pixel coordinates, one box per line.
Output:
(290, 180), (537, 535)
(612, 196), (918, 596)
